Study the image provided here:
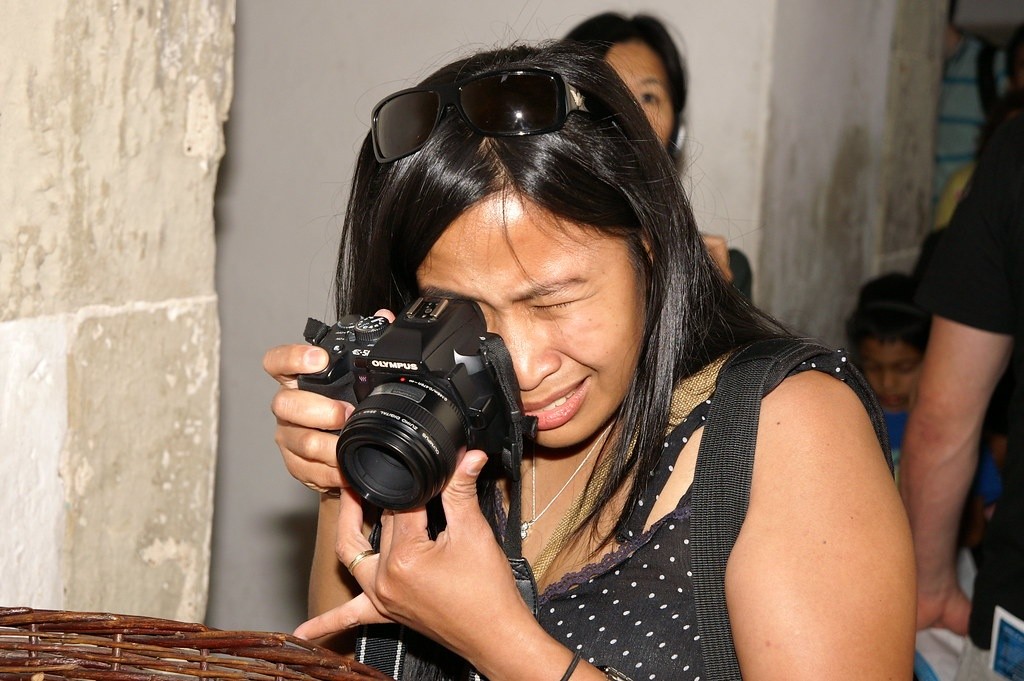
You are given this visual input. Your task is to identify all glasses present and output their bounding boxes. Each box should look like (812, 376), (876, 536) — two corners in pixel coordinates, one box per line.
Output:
(371, 65), (642, 163)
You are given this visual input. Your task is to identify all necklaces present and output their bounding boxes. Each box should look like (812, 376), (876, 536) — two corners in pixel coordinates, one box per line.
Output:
(521, 418), (616, 538)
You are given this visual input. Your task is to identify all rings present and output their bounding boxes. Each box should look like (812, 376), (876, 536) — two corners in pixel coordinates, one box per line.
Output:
(347, 549), (377, 576)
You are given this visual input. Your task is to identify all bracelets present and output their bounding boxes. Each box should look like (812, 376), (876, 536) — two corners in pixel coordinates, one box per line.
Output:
(558, 647), (582, 681)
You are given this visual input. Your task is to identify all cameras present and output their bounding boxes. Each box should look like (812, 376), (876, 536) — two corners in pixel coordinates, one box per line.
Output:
(295, 292), (538, 511)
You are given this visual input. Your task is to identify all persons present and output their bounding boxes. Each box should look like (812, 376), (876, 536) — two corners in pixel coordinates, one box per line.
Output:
(558, 11), (753, 305)
(845, 0), (1024, 680)
(255, 42), (915, 681)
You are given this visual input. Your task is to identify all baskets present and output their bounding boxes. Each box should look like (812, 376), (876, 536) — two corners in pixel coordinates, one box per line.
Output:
(0, 606), (391, 681)
